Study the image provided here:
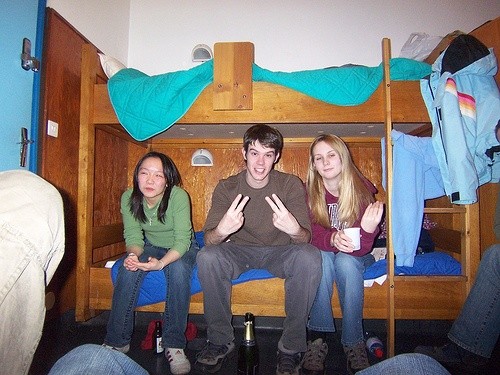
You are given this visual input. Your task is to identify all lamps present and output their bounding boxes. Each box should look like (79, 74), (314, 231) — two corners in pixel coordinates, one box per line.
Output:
(191, 45), (213, 63)
(191, 149), (213, 167)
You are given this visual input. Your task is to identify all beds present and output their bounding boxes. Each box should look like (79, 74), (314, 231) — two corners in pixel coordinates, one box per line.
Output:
(76, 30), (480, 359)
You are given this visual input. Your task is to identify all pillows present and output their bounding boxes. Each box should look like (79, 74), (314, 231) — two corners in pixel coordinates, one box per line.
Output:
(97, 53), (126, 79)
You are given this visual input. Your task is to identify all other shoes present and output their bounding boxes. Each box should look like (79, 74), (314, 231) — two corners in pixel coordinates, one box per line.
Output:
(414, 341), (477, 367)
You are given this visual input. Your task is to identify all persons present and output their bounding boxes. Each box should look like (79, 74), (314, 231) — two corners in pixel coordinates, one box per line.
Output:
(413, 243), (500, 370)
(194, 123), (322, 375)
(47, 344), (451, 375)
(302, 134), (383, 375)
(102, 152), (199, 375)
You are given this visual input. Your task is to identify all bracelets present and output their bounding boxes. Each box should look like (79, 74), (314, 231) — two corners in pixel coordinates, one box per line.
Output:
(159, 260), (164, 270)
(129, 253), (135, 256)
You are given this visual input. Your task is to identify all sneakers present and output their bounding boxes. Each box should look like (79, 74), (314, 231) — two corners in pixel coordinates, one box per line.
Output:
(343, 341), (369, 375)
(102, 343), (130, 354)
(164, 347), (192, 375)
(193, 341), (235, 373)
(275, 348), (301, 375)
(302, 338), (329, 375)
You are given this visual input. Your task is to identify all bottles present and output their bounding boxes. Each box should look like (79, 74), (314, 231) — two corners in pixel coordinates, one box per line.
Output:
(236, 311), (260, 375)
(363, 330), (384, 358)
(152, 321), (164, 357)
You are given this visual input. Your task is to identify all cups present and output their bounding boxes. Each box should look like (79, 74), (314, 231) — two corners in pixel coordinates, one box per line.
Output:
(343, 228), (361, 250)
